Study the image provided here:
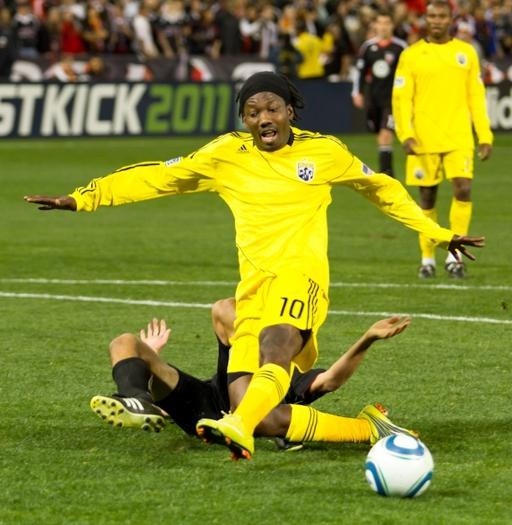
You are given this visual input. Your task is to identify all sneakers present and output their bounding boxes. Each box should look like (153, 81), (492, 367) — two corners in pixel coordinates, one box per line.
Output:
(273, 435), (304, 454)
(196, 413), (255, 460)
(356, 402), (419, 447)
(444, 261), (468, 280)
(416, 264), (436, 279)
(89, 393), (169, 435)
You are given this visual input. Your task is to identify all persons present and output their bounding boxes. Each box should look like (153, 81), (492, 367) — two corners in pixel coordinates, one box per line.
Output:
(24, 70), (484, 463)
(0, 1), (512, 81)
(388, 0), (495, 281)
(88, 295), (411, 434)
(350, 12), (409, 177)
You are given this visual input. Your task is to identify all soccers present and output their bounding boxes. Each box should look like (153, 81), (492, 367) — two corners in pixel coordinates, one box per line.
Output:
(365, 433), (434, 498)
